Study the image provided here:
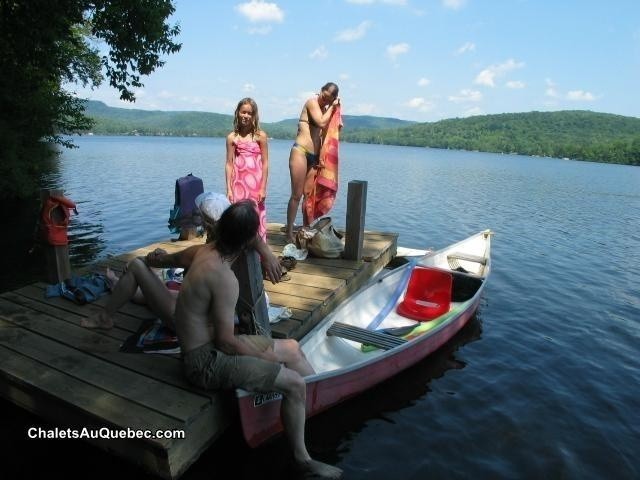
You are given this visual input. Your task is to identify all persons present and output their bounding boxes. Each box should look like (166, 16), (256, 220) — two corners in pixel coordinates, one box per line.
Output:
(174, 203), (344, 480)
(80, 191), (232, 333)
(225, 98), (268, 263)
(285, 83), (341, 244)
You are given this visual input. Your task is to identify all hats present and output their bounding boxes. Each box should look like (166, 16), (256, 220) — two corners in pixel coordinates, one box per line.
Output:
(195, 191), (232, 228)
(278, 243), (308, 261)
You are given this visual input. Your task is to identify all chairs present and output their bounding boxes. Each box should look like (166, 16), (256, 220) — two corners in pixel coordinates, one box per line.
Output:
(397, 267), (452, 321)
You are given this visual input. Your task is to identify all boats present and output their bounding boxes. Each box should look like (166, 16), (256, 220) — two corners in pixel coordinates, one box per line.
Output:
(236, 229), (493, 448)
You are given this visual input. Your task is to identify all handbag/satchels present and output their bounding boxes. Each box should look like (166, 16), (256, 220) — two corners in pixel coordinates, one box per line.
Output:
(297, 216), (345, 258)
